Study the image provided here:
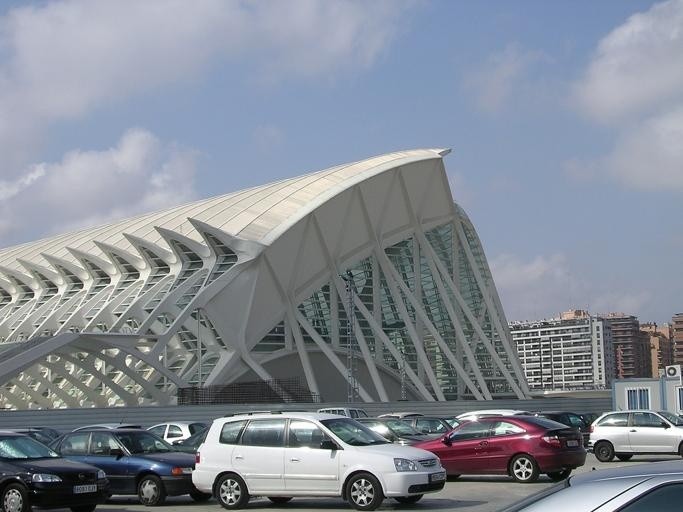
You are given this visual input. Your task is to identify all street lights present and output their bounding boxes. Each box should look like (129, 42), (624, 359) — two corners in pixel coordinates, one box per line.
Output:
(337, 269), (361, 402)
